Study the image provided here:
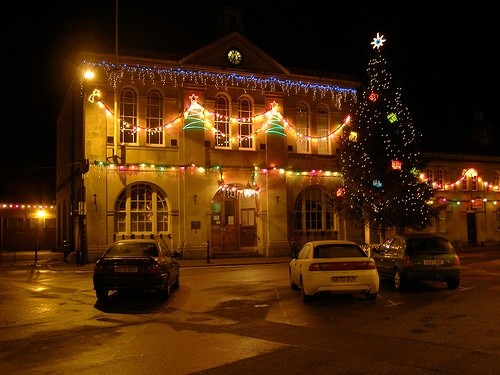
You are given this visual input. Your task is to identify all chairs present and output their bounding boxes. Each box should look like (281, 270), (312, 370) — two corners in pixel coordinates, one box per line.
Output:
(122, 246), (158, 257)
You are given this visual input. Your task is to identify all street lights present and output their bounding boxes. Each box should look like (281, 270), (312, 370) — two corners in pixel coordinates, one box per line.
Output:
(35, 208), (48, 261)
(80, 70), (94, 265)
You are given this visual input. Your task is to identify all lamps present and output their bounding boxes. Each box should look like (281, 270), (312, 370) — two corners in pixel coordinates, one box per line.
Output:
(242, 180), (253, 198)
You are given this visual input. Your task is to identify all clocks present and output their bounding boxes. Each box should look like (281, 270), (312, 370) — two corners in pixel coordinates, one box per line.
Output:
(226, 48), (242, 64)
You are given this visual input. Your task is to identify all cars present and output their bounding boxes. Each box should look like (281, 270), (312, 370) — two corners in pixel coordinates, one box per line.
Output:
(370, 233), (461, 290)
(289, 240), (379, 303)
(93, 239), (180, 301)
